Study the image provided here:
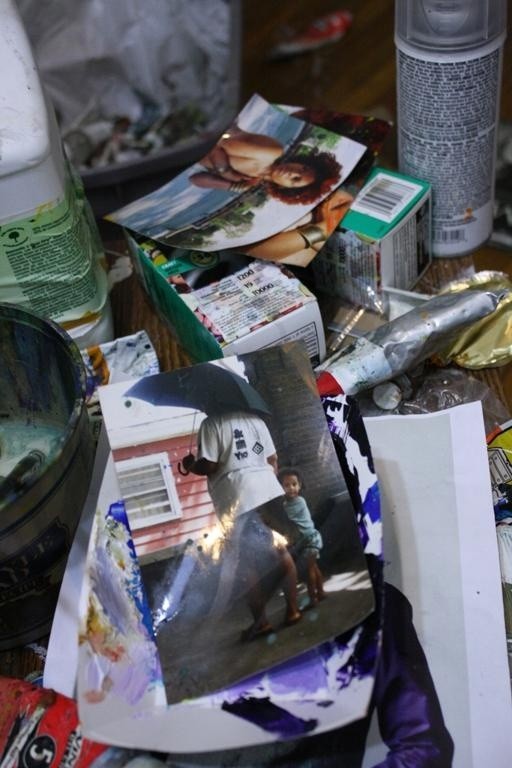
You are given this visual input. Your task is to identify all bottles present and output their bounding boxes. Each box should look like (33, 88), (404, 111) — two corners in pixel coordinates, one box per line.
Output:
(394, 0), (508, 261)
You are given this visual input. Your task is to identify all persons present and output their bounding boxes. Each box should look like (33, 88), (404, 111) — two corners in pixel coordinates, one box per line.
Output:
(277, 466), (327, 607)
(90, 117), (167, 167)
(189, 133), (344, 204)
(233, 191), (354, 259)
(295, 106), (392, 187)
(182, 410), (301, 641)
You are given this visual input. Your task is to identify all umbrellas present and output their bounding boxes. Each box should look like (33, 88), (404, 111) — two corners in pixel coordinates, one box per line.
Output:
(124, 362), (272, 476)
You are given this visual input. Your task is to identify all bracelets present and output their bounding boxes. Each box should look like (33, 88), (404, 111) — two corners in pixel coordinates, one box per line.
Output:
(212, 166), (230, 173)
(228, 180), (245, 194)
(299, 224), (326, 246)
